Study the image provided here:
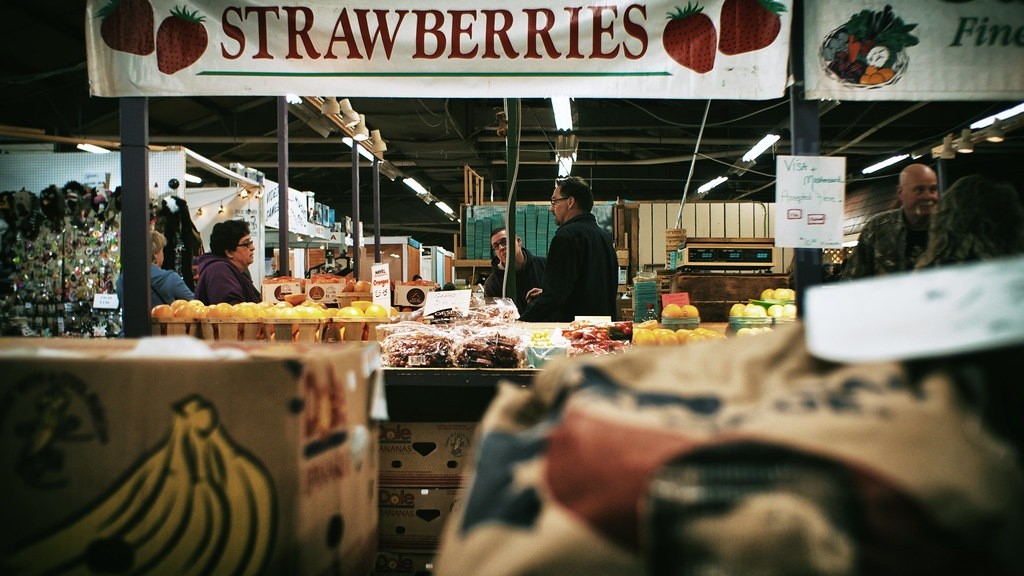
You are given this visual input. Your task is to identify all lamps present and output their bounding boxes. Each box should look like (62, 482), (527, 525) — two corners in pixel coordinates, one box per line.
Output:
(371, 160), (403, 182)
(726, 158), (757, 177)
(984, 118), (1006, 143)
(958, 129), (975, 154)
(558, 156), (572, 177)
(76, 143), (110, 154)
(218, 201), (225, 214)
(435, 201), (454, 215)
(320, 243), (326, 250)
(403, 178), (428, 195)
(940, 135), (956, 159)
(238, 188), (251, 199)
(697, 175), (729, 193)
(341, 137), (385, 165)
(185, 173), (203, 184)
(353, 114), (369, 142)
(371, 129), (387, 154)
(551, 96), (574, 132)
(197, 208), (202, 215)
(862, 148), (910, 176)
(296, 233), (305, 243)
(554, 134), (578, 163)
(741, 132), (781, 162)
(320, 97), (341, 115)
(969, 102), (1024, 130)
(338, 99), (361, 128)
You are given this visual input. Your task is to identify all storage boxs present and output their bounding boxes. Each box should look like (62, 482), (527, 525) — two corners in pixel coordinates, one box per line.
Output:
(151, 317), (200, 338)
(473, 293), (485, 299)
(332, 318), (390, 342)
(261, 282), (301, 304)
(393, 285), (435, 307)
(262, 318), (332, 343)
(617, 299), (632, 308)
(660, 317), (701, 332)
(528, 346), (568, 369)
(665, 229), (687, 270)
(0, 336), (379, 576)
(618, 266), (627, 284)
(621, 309), (633, 321)
(750, 298), (797, 311)
(375, 549), (437, 576)
(633, 281), (658, 324)
(305, 283), (346, 304)
(728, 316), (772, 333)
(336, 291), (373, 308)
(377, 422), (478, 490)
(771, 318), (797, 328)
(200, 316), (262, 341)
(617, 285), (629, 291)
(378, 487), (458, 550)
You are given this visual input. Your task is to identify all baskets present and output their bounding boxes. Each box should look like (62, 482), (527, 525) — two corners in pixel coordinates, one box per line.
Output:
(151, 313), (390, 346)
(729, 296), (797, 335)
(660, 313), (700, 330)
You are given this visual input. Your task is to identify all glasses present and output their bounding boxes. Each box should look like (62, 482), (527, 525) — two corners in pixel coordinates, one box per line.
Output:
(237, 241), (253, 249)
(492, 237), (506, 252)
(551, 196), (571, 206)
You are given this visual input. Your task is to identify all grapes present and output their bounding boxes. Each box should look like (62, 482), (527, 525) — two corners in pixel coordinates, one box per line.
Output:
(527, 330), (553, 347)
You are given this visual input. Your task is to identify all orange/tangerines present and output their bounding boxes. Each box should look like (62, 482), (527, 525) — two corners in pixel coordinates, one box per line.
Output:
(633, 304), (724, 345)
(729, 288), (797, 337)
(151, 298), (396, 342)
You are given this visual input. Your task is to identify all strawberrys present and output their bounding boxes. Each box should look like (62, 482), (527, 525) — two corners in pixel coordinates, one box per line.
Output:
(561, 321), (633, 357)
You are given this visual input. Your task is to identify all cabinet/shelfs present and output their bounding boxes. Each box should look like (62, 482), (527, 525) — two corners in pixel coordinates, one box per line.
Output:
(453, 232), (631, 321)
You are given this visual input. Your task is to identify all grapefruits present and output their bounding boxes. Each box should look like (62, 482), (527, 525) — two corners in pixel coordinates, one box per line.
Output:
(285, 281), (371, 305)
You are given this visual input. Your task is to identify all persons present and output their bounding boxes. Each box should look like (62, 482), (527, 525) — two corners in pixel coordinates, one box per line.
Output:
(116, 231), (195, 338)
(474, 272), (489, 286)
(194, 220), (262, 307)
(823, 263), (841, 283)
(840, 164), (990, 283)
(413, 275), (422, 282)
(518, 176), (618, 322)
(484, 227), (546, 315)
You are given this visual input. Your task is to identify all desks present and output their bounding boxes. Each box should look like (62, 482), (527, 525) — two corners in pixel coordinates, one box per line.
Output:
(383, 366), (547, 392)
